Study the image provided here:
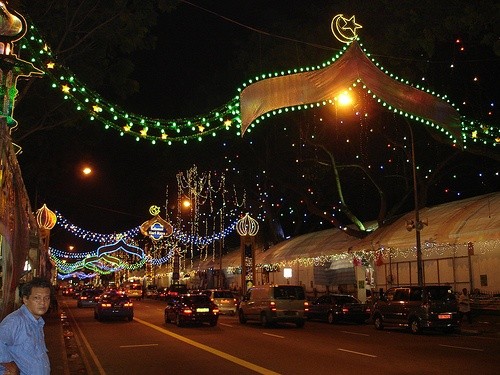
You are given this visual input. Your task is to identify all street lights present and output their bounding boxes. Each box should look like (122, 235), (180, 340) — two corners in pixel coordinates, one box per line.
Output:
(332, 88), (428, 287)
(182, 199), (223, 288)
(35, 166), (92, 278)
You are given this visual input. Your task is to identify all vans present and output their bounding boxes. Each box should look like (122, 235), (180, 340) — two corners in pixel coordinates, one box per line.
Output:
(373, 285), (460, 335)
(237, 284), (307, 329)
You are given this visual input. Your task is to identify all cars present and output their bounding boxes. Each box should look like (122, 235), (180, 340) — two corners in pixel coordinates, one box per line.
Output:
(208, 288), (236, 316)
(94, 292), (134, 321)
(104, 282), (212, 302)
(164, 294), (218, 327)
(77, 288), (104, 308)
(305, 294), (371, 324)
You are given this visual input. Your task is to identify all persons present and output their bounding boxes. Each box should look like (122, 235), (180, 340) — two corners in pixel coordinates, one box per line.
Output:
(459, 288), (472, 324)
(0, 278), (54, 375)
